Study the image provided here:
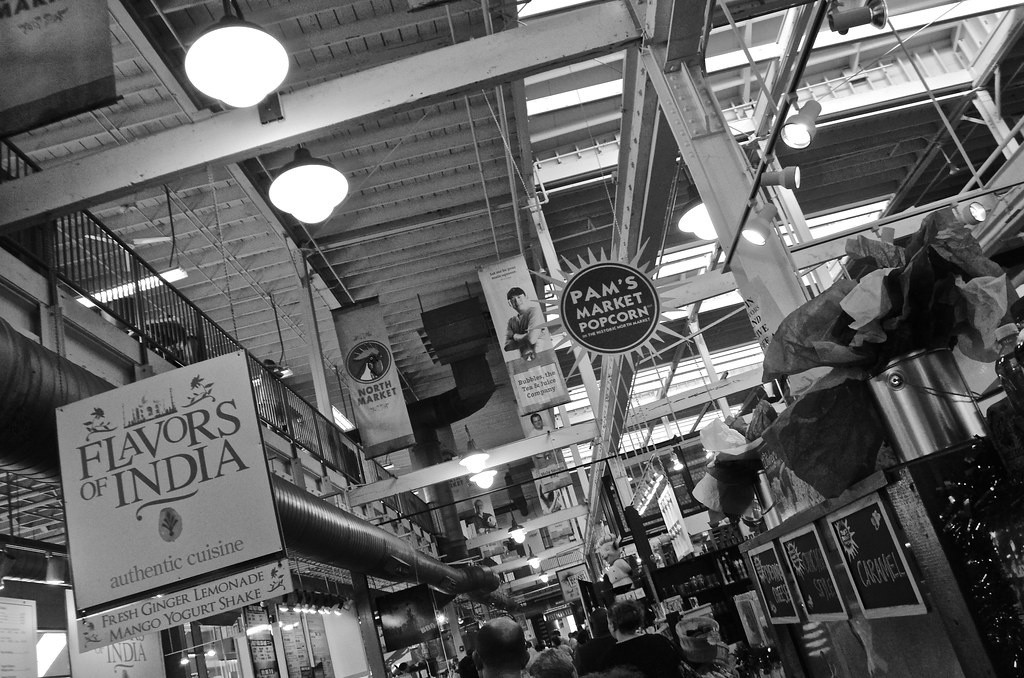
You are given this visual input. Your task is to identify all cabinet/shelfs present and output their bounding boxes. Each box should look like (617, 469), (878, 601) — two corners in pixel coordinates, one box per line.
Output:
(650, 541), (755, 645)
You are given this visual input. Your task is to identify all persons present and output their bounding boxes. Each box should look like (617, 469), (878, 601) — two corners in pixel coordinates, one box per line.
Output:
(598, 599), (682, 678)
(260, 360), (289, 433)
(473, 499), (496, 529)
(503, 288), (547, 356)
(455, 650), (479, 678)
(574, 607), (618, 678)
(527, 629), (590, 656)
(472, 616), (530, 678)
(520, 646), (578, 678)
(538, 482), (561, 511)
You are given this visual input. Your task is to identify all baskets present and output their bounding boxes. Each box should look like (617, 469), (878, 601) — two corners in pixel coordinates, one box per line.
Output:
(675, 616), (718, 664)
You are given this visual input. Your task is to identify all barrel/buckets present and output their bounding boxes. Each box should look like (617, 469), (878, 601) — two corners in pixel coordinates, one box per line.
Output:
(676, 618), (720, 663)
(863, 344), (989, 462)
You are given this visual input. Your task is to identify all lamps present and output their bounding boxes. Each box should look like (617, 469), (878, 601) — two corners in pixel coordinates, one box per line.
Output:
(251, 303), (293, 386)
(468, 469), (498, 489)
(332, 365), (356, 432)
(963, 203), (987, 223)
(279, 601), (289, 613)
(761, 166), (801, 189)
(538, 567), (549, 582)
(669, 448), (683, 471)
(741, 198), (776, 246)
(526, 545), (540, 569)
(180, 652), (189, 665)
(938, 147), (961, 174)
(826, 0), (889, 35)
(184, 0), (290, 108)
(293, 600), (353, 616)
(676, 165), (719, 239)
(207, 643), (216, 656)
(459, 425), (490, 474)
(632, 463), (664, 515)
(74, 184), (188, 308)
(269, 143), (349, 224)
(507, 506), (527, 544)
(780, 92), (822, 150)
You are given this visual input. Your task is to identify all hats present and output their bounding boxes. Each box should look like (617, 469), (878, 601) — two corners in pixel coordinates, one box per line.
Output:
(474, 500), (483, 507)
(505, 287), (526, 301)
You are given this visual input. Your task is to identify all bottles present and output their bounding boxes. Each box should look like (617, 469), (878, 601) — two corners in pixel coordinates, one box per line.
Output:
(652, 573), (720, 619)
(994, 296), (1024, 423)
(732, 641), (787, 678)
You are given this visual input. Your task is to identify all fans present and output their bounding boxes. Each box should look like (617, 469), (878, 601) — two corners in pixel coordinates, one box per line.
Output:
(86, 207), (171, 272)
